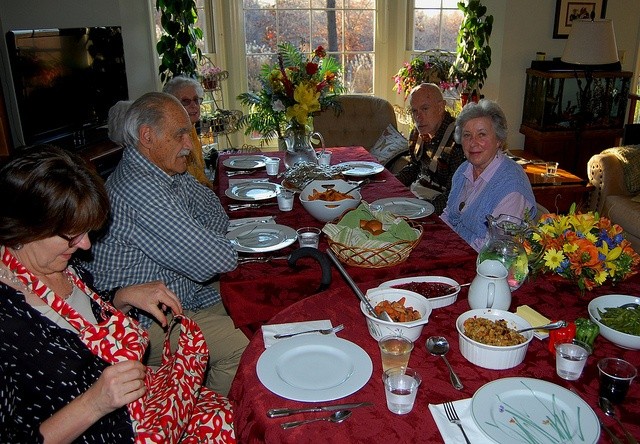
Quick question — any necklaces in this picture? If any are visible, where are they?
[0,269,74,298]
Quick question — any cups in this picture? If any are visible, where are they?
[265,157,281,176]
[316,151,333,165]
[277,189,295,212]
[295,226,321,249]
[553,338,592,381]
[378,335,415,376]
[546,161,559,177]
[381,366,423,416]
[597,358,638,416]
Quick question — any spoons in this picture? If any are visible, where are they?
[323,248,392,323]
[280,410,353,430]
[425,335,464,390]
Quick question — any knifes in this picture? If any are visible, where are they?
[239,257,289,261]
[267,402,364,419]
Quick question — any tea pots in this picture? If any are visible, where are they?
[475,214,548,293]
[467,258,512,310]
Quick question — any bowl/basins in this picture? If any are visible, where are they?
[455,308,534,370]
[587,294,640,351]
[359,288,433,342]
[300,179,362,224]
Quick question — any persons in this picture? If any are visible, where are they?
[394,82,464,214]
[0,146,184,444]
[162,77,213,189]
[70,93,251,398]
[439,100,537,252]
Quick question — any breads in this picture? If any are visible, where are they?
[359,219,385,235]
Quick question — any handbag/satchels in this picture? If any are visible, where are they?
[134,315,210,444]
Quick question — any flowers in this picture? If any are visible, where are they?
[395,48,469,100]
[202,67,221,77]
[519,203,640,291]
[235,39,341,146]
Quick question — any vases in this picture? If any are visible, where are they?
[202,76,218,89]
[282,120,323,169]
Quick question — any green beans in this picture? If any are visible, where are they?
[595,303,640,336]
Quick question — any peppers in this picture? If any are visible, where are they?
[547,319,577,357]
[574,317,600,352]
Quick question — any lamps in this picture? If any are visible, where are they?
[561,18,619,66]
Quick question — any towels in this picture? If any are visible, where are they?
[261,320,334,348]
[229,178,271,187]
[226,216,277,230]
[428,398,492,444]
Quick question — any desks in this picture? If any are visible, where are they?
[507,149,595,213]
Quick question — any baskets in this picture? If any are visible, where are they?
[325,208,423,268]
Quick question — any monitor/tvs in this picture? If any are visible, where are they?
[0,25,129,156]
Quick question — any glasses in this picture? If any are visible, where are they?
[57,223,97,249]
[181,98,203,107]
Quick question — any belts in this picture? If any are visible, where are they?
[418,177,446,193]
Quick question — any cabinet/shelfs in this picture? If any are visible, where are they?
[42,130,125,181]
[520,125,620,176]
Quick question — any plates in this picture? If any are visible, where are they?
[377,275,459,308]
[222,155,272,170]
[225,223,298,253]
[255,334,374,403]
[281,177,301,193]
[471,377,601,444]
[224,181,283,201]
[338,160,385,177]
[369,196,435,220]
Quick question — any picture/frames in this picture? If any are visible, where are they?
[552,0,606,39]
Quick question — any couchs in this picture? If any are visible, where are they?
[586,144,639,249]
[309,94,416,187]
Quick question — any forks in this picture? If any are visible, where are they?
[238,258,273,264]
[274,324,345,339]
[442,400,474,444]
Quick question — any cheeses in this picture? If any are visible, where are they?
[516,304,551,333]
